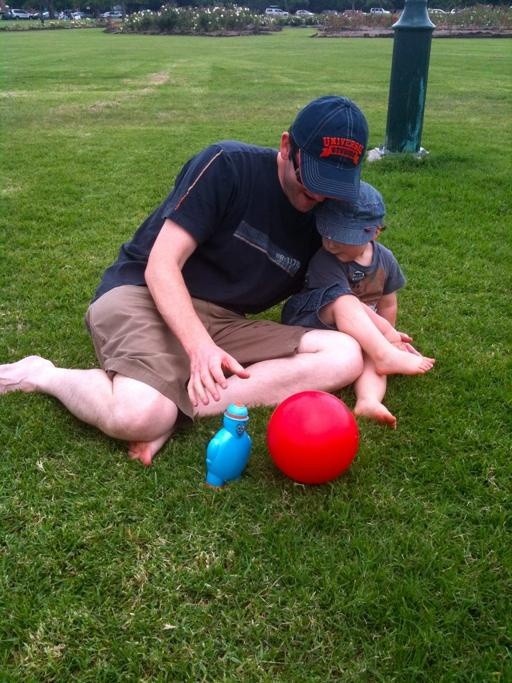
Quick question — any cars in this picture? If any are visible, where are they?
[31,9,123,21]
[427,8,461,15]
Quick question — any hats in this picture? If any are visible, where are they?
[317,180,385,246]
[290,95,370,202]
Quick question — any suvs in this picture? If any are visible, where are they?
[4,9,33,20]
[264,8,392,18]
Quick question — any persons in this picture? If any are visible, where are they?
[277,177,437,432]
[1,90,414,471]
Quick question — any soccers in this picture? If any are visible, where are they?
[267,390,360,484]
